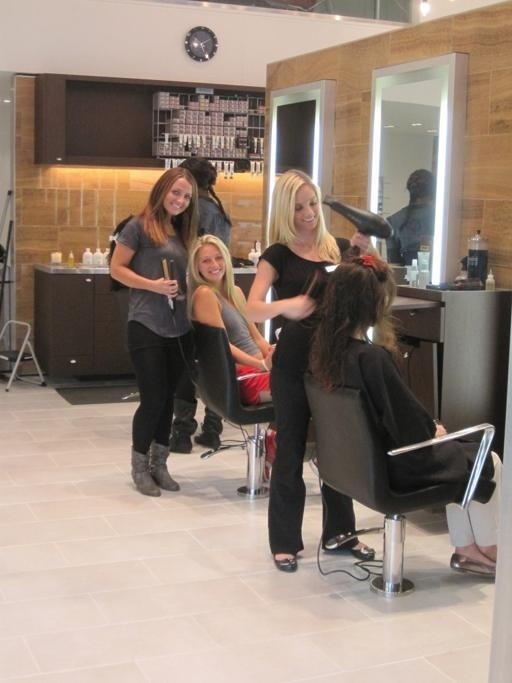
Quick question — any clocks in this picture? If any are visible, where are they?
[185,26,219,62]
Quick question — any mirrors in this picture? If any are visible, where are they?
[365,52,470,286]
[266,78,336,237]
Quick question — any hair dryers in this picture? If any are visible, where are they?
[323,193,395,240]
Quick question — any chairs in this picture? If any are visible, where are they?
[190,320,275,497]
[0,320,45,391]
[304,373,496,597]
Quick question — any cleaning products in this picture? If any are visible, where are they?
[417,251,431,282]
[82,247,110,266]
[67,250,75,268]
[467,230,487,289]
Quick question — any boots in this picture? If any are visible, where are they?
[169,396,197,453]
[149,440,181,490]
[194,407,223,451]
[131,447,164,496]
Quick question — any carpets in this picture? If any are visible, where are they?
[55,385,141,405]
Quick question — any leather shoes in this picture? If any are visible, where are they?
[449,553,496,575]
[349,542,376,561]
[273,554,296,572]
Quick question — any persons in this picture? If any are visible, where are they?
[385,169,434,264]
[168,157,231,453]
[110,168,199,496]
[187,233,277,480]
[246,169,382,572]
[308,255,503,580]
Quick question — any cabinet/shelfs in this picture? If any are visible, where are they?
[152,93,265,160]
[383,283,512,455]
[34,263,263,376]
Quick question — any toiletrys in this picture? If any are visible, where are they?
[50,251,63,264]
